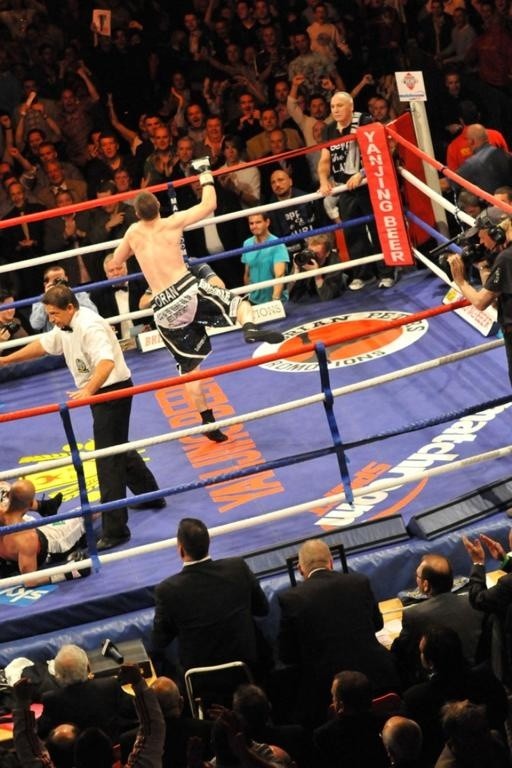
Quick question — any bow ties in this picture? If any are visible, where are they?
[112,284,129,292]
[60,325,74,333]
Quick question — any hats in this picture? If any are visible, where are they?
[465,206,510,239]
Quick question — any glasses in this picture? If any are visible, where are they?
[416,573,421,579]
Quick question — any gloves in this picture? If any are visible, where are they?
[49,550,93,585]
[188,155,215,187]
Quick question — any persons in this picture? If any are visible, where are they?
[286,231,343,302]
[0,289,29,342]
[447,208,512,392]
[233,686,312,768]
[461,528,512,753]
[0,91,84,220]
[185,704,295,767]
[119,676,215,768]
[0,481,102,588]
[0,284,167,550]
[457,189,488,218]
[30,266,99,332]
[45,724,80,768]
[138,257,225,310]
[367,95,383,114]
[269,169,315,237]
[12,663,167,768]
[306,120,326,183]
[371,98,392,126]
[115,155,284,443]
[38,643,140,742]
[398,626,510,748]
[241,213,289,306]
[317,92,402,291]
[277,539,384,668]
[0,182,48,293]
[0,0,407,91]
[381,716,423,768]
[312,672,390,768]
[149,518,269,669]
[286,73,374,147]
[390,554,483,662]
[91,254,156,340]
[41,190,96,287]
[84,91,294,200]
[493,186,512,214]
[87,180,138,312]
[433,700,512,768]
[407,0,512,193]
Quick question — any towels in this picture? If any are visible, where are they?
[343,111,363,175]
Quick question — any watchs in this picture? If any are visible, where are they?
[478,265,490,271]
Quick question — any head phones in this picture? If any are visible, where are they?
[480,208,507,245]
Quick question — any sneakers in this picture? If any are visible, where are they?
[39,491,64,518]
[129,496,168,511]
[347,274,379,291]
[95,527,132,551]
[198,421,228,443]
[377,266,401,290]
[242,329,286,344]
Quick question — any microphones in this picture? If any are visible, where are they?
[100,638,125,662]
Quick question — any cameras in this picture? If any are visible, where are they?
[2,321,23,337]
[53,277,69,290]
[428,226,488,275]
[293,249,319,268]
